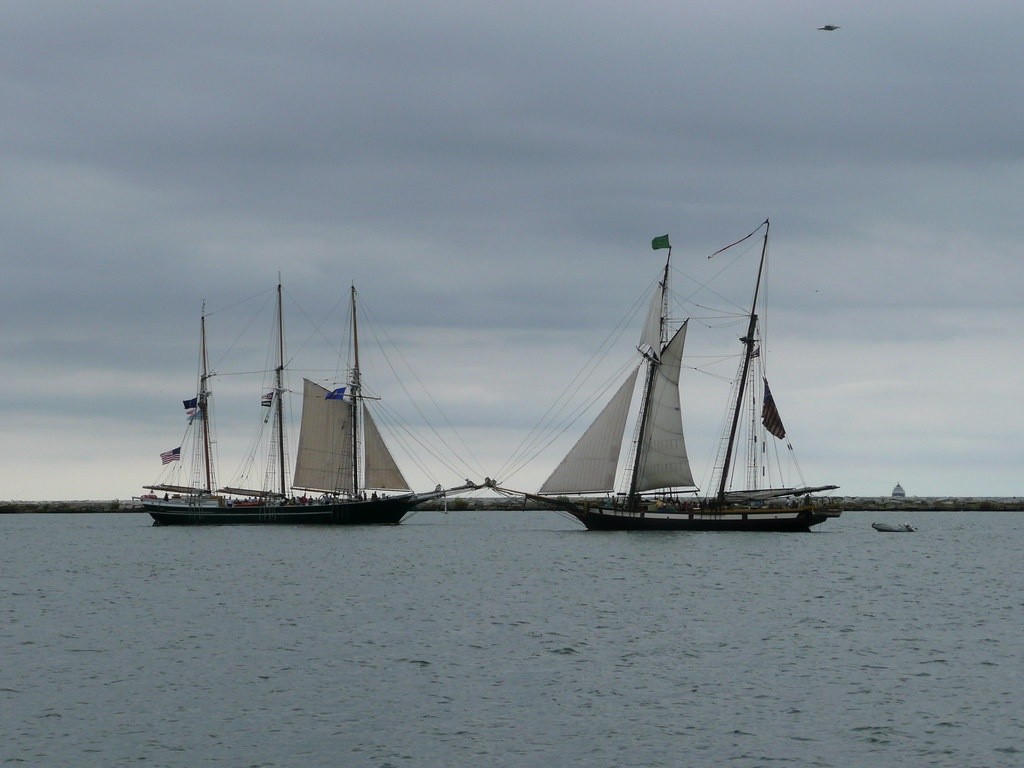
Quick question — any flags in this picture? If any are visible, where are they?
[760,377,786,440]
[261,391,273,407]
[160,447,181,464]
[325,387,347,401]
[651,234,671,250]
[183,398,205,421]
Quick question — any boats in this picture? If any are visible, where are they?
[871,519,919,533]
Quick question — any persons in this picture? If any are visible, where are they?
[583,495,639,514]
[653,494,718,511]
[226,491,378,507]
[786,493,816,508]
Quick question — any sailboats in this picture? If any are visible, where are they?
[130,269,490,527]
[491,219,845,534]
